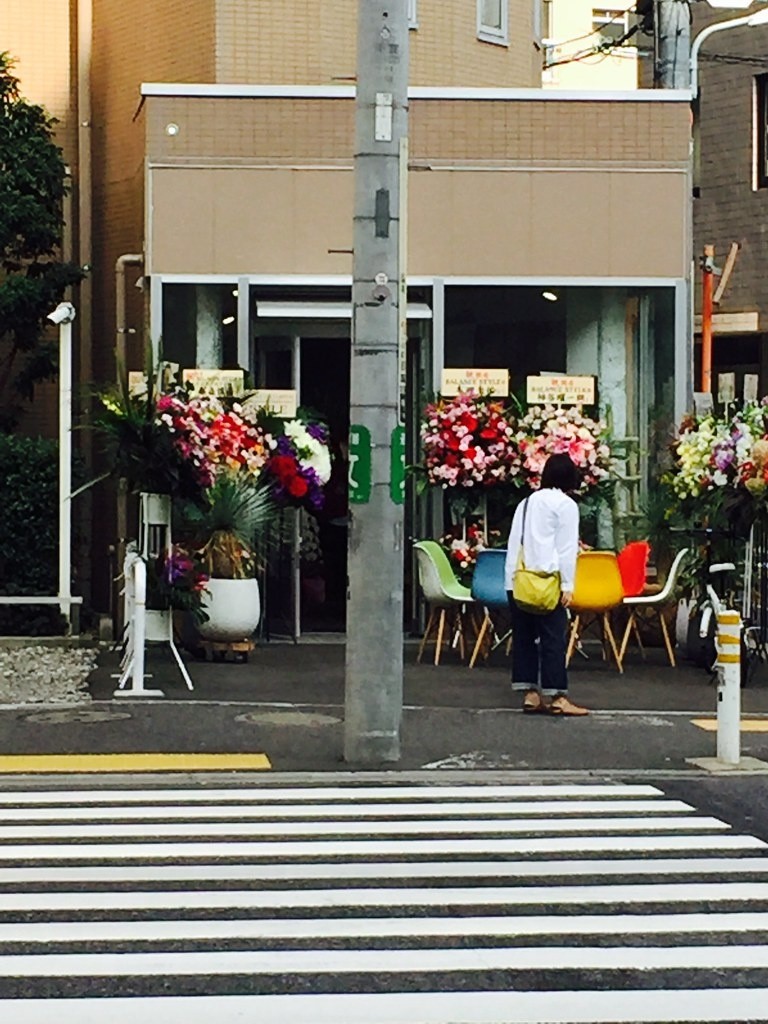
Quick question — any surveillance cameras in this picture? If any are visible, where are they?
[46,305,71,326]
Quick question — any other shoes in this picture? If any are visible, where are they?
[523,691,590,715]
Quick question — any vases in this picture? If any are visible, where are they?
[144,610,171,640]
[676,598,749,690]
[143,494,168,525]
[192,577,260,642]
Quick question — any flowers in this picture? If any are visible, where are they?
[66,336,332,628]
[660,396,768,596]
[419,386,611,570]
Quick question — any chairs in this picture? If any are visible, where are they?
[616,540,650,659]
[619,548,689,667]
[468,549,510,671]
[412,541,487,666]
[565,551,625,674]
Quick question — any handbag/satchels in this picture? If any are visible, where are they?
[512,545,562,615]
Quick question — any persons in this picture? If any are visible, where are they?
[504,453,593,716]
